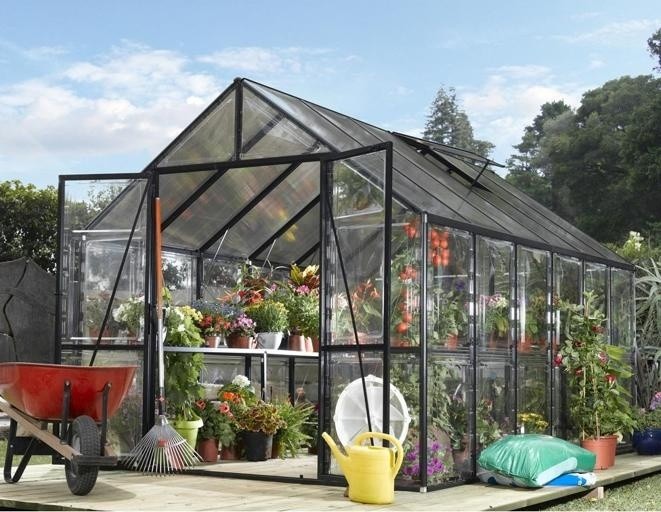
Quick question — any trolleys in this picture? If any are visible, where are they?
[0,362,141,495]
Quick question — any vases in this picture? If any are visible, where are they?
[193,430,289,460]
[631,427,661,457]
[580,437,619,467]
[203,332,368,358]
[89,329,141,347]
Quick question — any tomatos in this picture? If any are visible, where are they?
[394,217,449,333]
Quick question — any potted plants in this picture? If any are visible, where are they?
[154,305,204,465]
[428,289,571,353]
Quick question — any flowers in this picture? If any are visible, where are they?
[630,392,661,426]
[195,374,287,429]
[399,434,449,487]
[86,261,382,333]
[550,288,623,435]
